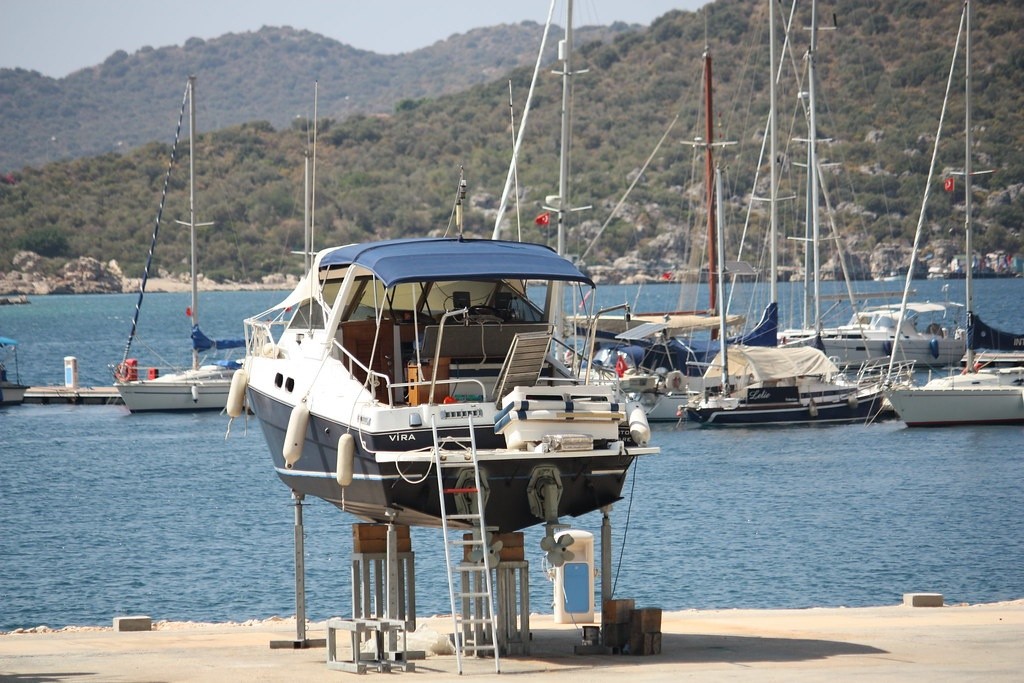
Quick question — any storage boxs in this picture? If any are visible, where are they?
[602,597,662,656]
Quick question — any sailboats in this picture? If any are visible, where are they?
[463,0,897,426]
[886,0,1024,424]
[108,75,293,412]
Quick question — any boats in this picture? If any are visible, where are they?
[244,166,661,535]
[779,296,965,366]
[0,337,30,404]
